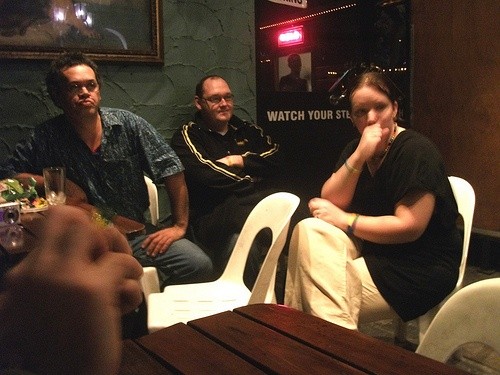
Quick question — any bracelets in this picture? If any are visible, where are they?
[226,155,231,167]
[347,212,359,235]
[344,160,362,174]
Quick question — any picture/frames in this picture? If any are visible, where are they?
[0,0,164,63]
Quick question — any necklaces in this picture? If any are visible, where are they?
[378,121,397,158]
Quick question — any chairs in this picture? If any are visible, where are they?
[415,278,500,363]
[394,175,476,347]
[147,192,300,334]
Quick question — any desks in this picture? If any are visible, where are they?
[118,303,474,375]
[0,194,146,268]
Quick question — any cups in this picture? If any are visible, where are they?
[42,167,67,206]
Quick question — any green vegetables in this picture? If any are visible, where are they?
[2,177,37,202]
[100,207,117,223]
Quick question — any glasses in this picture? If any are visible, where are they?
[200,93,235,105]
[59,81,99,95]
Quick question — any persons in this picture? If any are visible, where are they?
[171,75,303,304]
[0,204,144,375]
[284,72,464,332]
[0,53,213,293]
